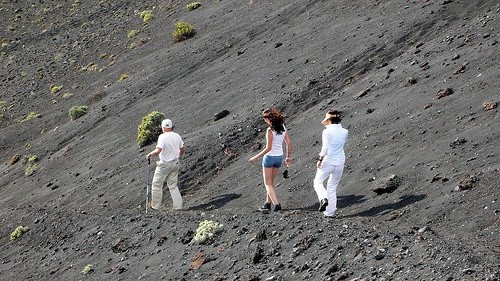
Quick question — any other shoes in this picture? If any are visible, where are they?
[324,214,335,218]
[148,202,152,208]
[318,198,328,212]
[172,208,182,211]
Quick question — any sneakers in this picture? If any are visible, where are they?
[273,204,283,213]
[259,202,271,211]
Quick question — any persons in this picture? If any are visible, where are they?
[146,119,184,211]
[313,110,349,217]
[248,107,291,212]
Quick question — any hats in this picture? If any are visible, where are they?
[161,119,172,128]
[321,109,340,125]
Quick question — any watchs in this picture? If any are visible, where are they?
[316,158,322,161]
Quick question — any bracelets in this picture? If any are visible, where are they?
[256,154,259,159]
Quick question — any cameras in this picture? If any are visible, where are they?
[283,170,288,178]
